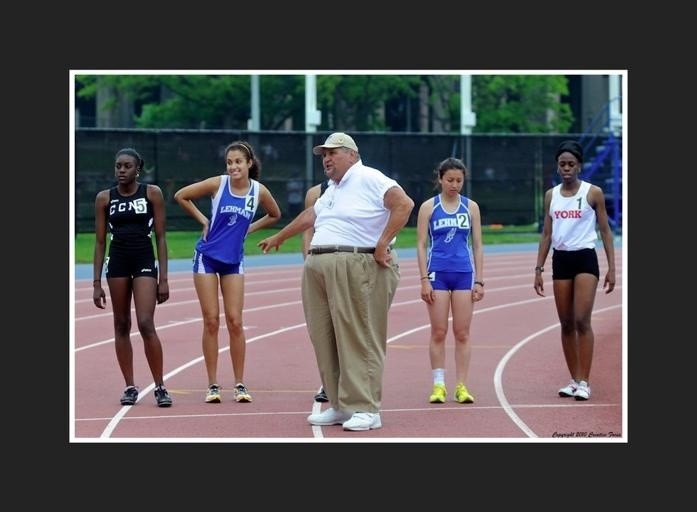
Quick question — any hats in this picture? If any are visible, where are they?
[312,133,358,153]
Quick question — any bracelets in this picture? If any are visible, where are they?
[419,276,431,281]
[93,279,101,283]
[473,280,483,287]
[535,265,544,274]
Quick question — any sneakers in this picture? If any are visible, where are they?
[120,386,139,405]
[429,383,447,403]
[233,384,252,402]
[307,406,354,430]
[314,386,329,402]
[453,383,474,403]
[343,411,383,430]
[204,383,222,403]
[558,379,593,400]
[154,385,172,407]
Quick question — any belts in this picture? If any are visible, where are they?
[307,245,394,254]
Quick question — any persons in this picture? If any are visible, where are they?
[174,139,280,405]
[532,146,615,400]
[91,144,172,408]
[256,132,413,431]
[416,156,483,403]
[302,179,331,407]
